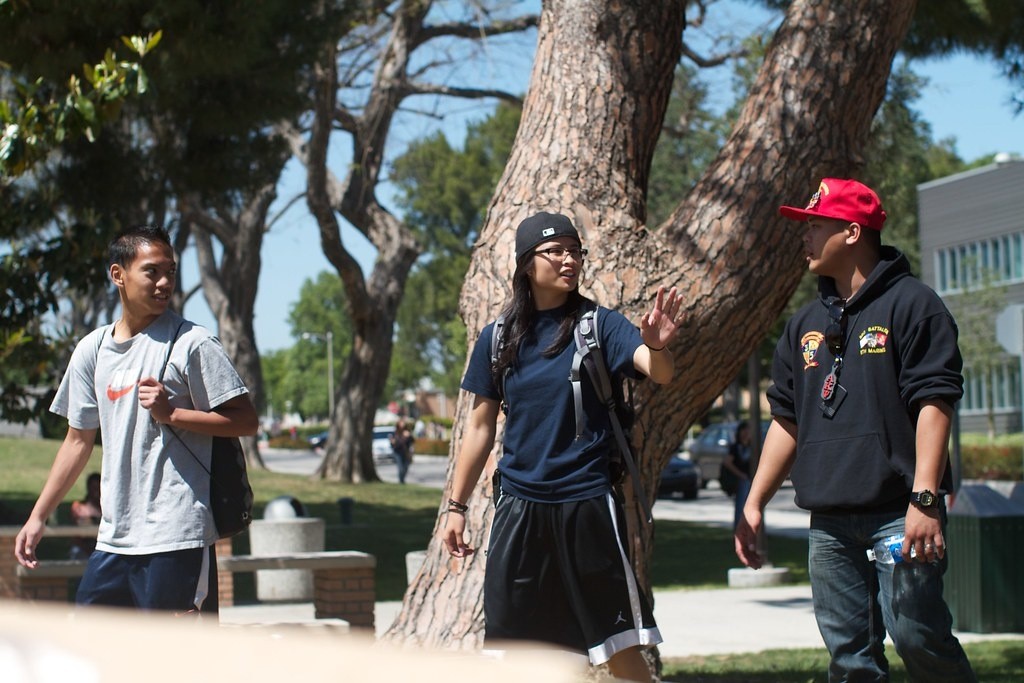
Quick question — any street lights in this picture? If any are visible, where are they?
[301,330,336,429]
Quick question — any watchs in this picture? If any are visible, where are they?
[911,490,940,509]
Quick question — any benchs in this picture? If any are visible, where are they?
[0,527,234,607]
[16,550,376,638]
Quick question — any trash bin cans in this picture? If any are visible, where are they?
[939,483,1024,632]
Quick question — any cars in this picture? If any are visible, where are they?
[655,455,701,500]
[371,425,415,464]
[687,420,771,490]
[306,429,329,452]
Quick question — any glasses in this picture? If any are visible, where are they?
[824,299,847,353]
[533,245,587,263]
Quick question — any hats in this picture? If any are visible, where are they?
[515,211,582,261]
[779,179,888,232]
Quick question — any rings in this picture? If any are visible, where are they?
[924,544,933,549]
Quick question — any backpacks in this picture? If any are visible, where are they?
[158,321,254,539]
[718,444,743,495]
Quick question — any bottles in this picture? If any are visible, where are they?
[866,534,944,565]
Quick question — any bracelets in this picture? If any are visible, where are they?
[448,498,468,513]
[645,342,665,351]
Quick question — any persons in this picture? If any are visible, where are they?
[724,419,751,525]
[388,419,415,483]
[735,178,973,683]
[15,227,259,616]
[443,211,684,683]
